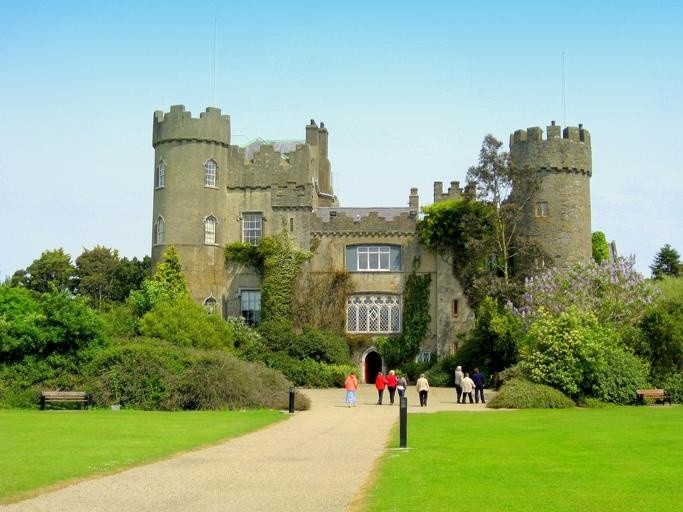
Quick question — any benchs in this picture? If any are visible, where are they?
[635,388,673,406]
[39,390,93,411]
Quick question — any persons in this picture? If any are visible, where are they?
[472,368,486,404]
[462,373,476,404]
[415,373,430,407]
[344,371,358,408]
[387,370,407,405]
[454,365,464,404]
[375,372,388,405]
